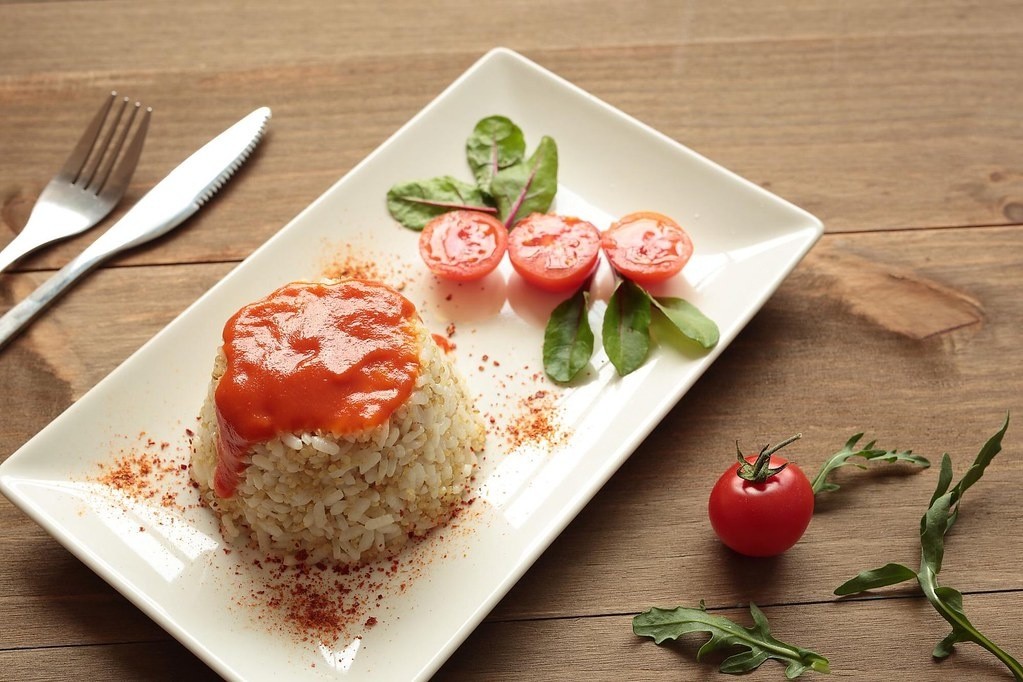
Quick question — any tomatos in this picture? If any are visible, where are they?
[599,209,694,286]
[707,435,816,558]
[507,214,600,291]
[420,209,508,284]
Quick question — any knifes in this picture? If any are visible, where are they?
[0,103,274,357]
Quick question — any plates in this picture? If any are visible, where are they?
[1,44,823,682]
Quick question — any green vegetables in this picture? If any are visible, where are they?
[631,599,829,679]
[542,280,719,382]
[832,413,1023,682]
[386,115,557,233]
[813,431,928,505]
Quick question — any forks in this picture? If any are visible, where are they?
[0,89,154,281]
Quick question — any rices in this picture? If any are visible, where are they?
[189,280,487,568]
